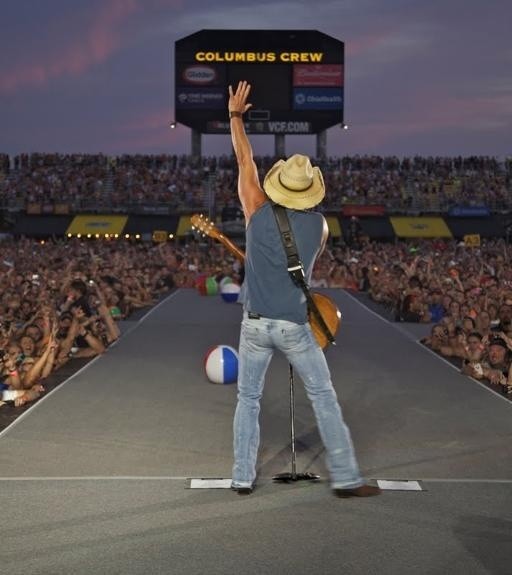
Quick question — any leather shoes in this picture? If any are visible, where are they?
[336,485,381,498]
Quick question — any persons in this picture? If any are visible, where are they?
[226,80,384,499]
[1,152,246,409]
[253,154,510,390]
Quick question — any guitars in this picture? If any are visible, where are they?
[190,214,341,353]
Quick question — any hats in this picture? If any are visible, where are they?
[262,153,326,211]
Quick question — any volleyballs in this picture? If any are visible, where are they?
[204,345,239,384]
[199,278,217,296]
[222,283,241,302]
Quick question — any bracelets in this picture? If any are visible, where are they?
[11,370,17,376]
[83,330,89,338]
[227,109,245,121]
[464,346,469,351]
[10,368,16,372]
[479,344,484,350]
